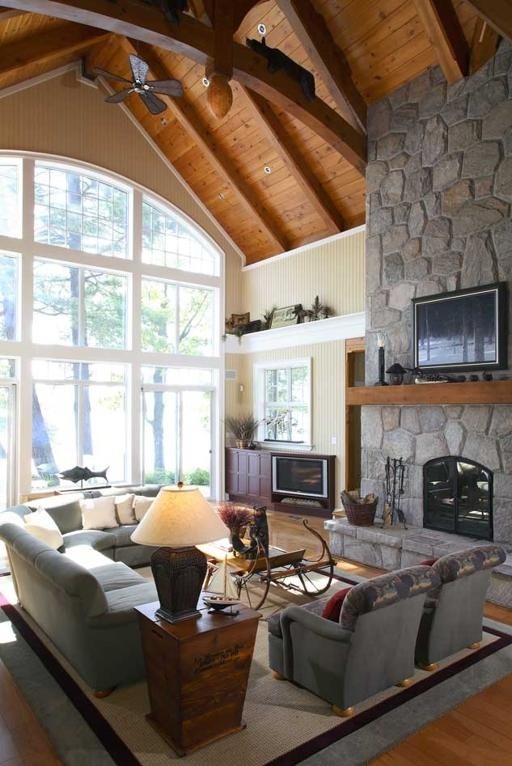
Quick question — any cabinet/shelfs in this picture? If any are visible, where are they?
[248,449,273,506]
[226,448,247,510]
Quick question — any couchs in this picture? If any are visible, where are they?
[1,484,176,697]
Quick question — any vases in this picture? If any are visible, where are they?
[229,526,241,544]
[234,439,248,449]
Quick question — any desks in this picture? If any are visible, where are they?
[135,590,263,755]
[190,517,338,610]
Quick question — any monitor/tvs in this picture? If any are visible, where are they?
[410,280,509,375]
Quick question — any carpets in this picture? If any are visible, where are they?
[1,557,512,766]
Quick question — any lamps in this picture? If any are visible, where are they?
[132,480,233,623]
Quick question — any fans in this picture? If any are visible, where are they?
[85,41,184,114]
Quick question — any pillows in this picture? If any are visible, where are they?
[80,496,120,530]
[25,504,43,522]
[113,493,139,524]
[131,495,155,524]
[320,587,353,622]
[27,509,64,550]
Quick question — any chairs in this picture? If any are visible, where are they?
[268,567,440,717]
[411,545,507,671]
